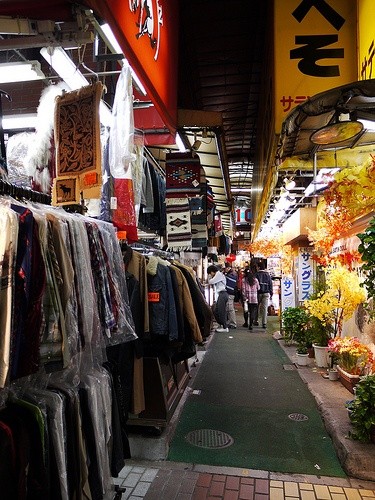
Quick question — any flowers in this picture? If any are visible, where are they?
[326,335,375,377]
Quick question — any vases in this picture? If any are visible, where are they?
[326,369,338,382]
[335,364,364,395]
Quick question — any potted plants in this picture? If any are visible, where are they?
[343,373,375,444]
[281,271,337,368]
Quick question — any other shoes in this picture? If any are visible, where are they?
[230,324,235,328]
[249,326,253,330]
[215,325,229,333]
[242,323,248,327]
[262,324,266,328]
[253,322,258,325]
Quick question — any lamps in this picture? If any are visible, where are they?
[283,177,296,190]
[193,131,202,150]
[280,186,289,198]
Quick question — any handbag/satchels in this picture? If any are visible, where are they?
[234,286,241,302]
[243,299,250,313]
[267,299,275,315]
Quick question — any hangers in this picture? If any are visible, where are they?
[0,57,177,401]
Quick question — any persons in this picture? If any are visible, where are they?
[234,268,242,304]
[242,262,274,330]
[207,265,229,332]
[224,264,237,328]
